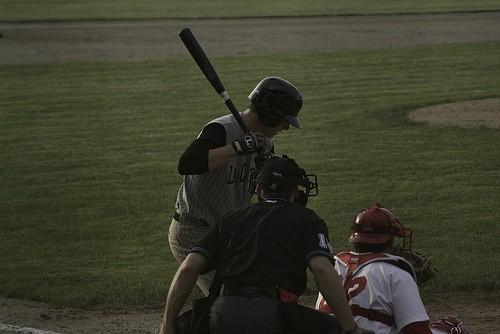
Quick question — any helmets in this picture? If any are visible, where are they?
[248,76,304,129]
[347,206,406,244]
[253,155,318,206]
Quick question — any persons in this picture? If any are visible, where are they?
[169,77,303,296]
[159,157,378,334]
[314,207,464,334]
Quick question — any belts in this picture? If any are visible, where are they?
[172,211,206,225]
[222,285,274,297]
[232,132,276,158]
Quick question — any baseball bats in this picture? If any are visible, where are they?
[177,27,264,162]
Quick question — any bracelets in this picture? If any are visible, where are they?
[344,324,358,334]
